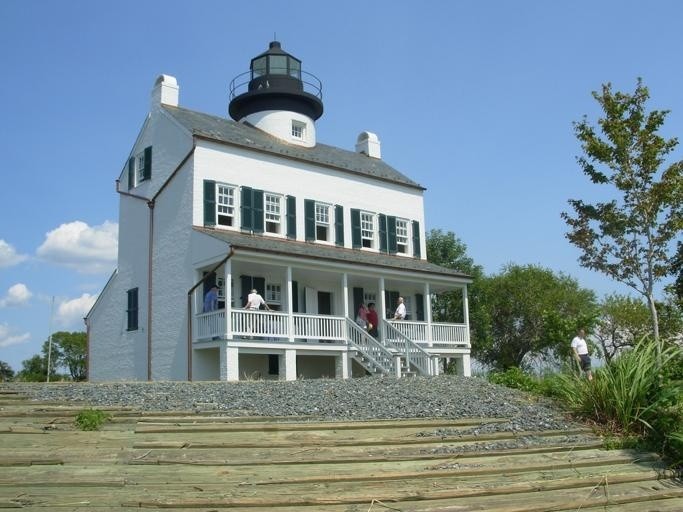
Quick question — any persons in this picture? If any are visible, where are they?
[388,296,406,321]
[568,328,593,381]
[202,284,226,342]
[241,288,270,340]
[355,302,369,331]
[366,302,379,341]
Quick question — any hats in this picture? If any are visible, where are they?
[210,285,220,289]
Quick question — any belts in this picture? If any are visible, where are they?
[249,307,259,310]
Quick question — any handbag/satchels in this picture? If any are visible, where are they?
[356,313,367,326]
